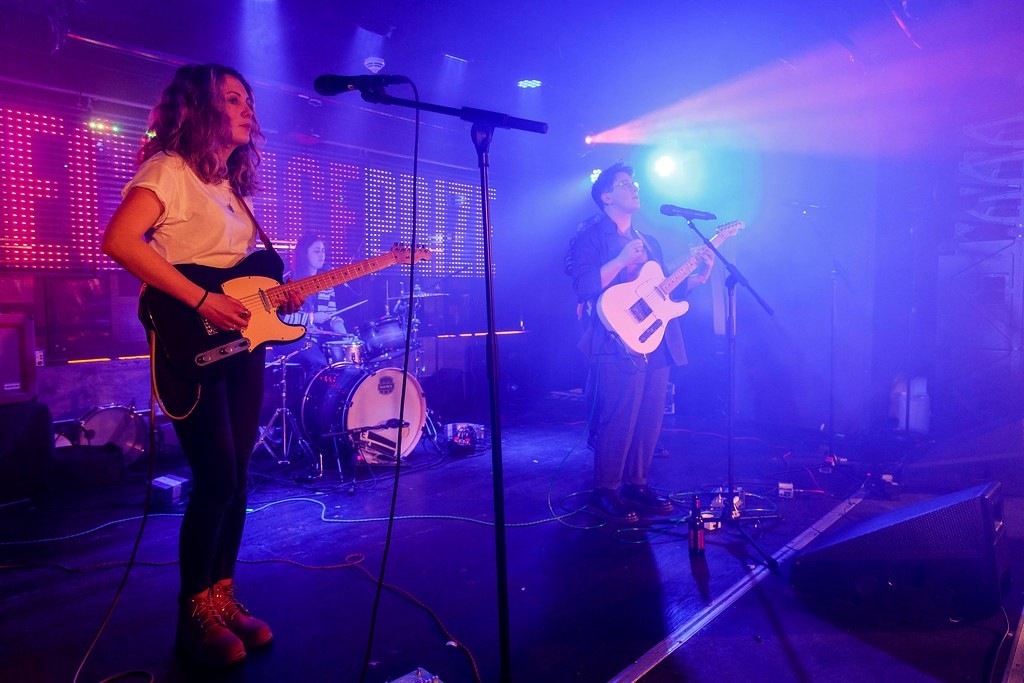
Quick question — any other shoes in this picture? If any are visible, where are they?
[622,486,673,513]
[654,448,670,458]
[585,436,597,451]
[584,487,639,523]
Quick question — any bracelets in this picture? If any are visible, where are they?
[194,290,208,310]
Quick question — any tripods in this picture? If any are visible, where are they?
[251,332,321,475]
[782,211,879,496]
[639,218,782,569]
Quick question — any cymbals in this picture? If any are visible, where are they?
[305,328,344,336]
[386,291,451,300]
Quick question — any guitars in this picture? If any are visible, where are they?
[595,219,745,358]
[136,241,433,379]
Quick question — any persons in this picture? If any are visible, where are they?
[281,234,348,379]
[563,159,714,524]
[99,62,307,660]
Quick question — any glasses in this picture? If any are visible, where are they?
[607,180,640,193]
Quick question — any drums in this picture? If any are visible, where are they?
[322,339,367,364]
[352,315,421,363]
[68,401,149,470]
[301,361,427,465]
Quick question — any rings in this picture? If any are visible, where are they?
[228,323,237,330]
[241,309,248,317]
[635,248,640,253]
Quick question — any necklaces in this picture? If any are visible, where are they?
[215,181,235,213]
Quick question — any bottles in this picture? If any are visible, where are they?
[688,494,705,556]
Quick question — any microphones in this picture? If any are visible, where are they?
[314,73,409,96]
[660,204,717,220]
[793,201,825,209]
[386,418,410,428]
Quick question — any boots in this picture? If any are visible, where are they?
[181,586,245,664]
[213,578,273,645]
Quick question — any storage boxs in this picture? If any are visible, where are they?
[663,383,677,415]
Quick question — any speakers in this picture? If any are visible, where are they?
[795,479,1010,633]
[889,411,1024,499]
[0,313,39,405]
